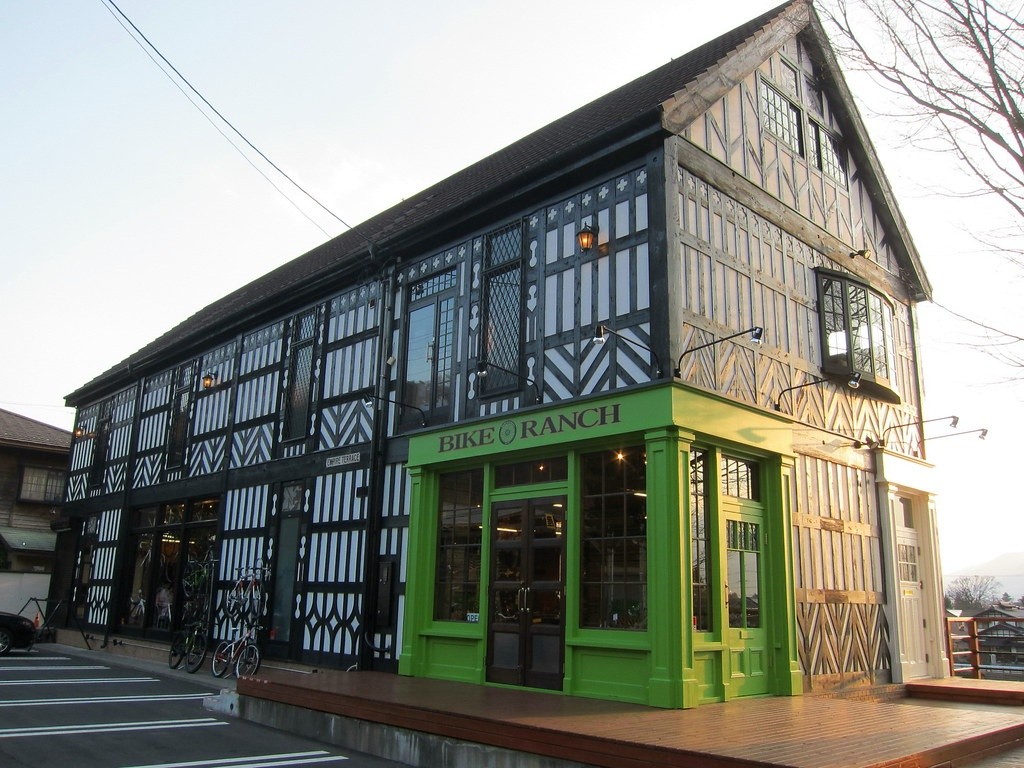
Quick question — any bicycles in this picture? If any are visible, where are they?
[212,623,271,679]
[128,538,205,626]
[168,618,213,673]
[225,566,262,620]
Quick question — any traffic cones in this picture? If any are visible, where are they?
[34,613,40,627]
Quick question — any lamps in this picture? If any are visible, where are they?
[914,428,987,449]
[850,249,872,259]
[592,323,660,381]
[854,436,880,450]
[202,372,215,389]
[576,220,596,253]
[776,372,862,411]
[883,416,962,447]
[361,391,428,427]
[677,326,764,378]
[477,359,544,407]
[74,428,83,439]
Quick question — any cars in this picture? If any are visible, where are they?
[0,612,36,656]
